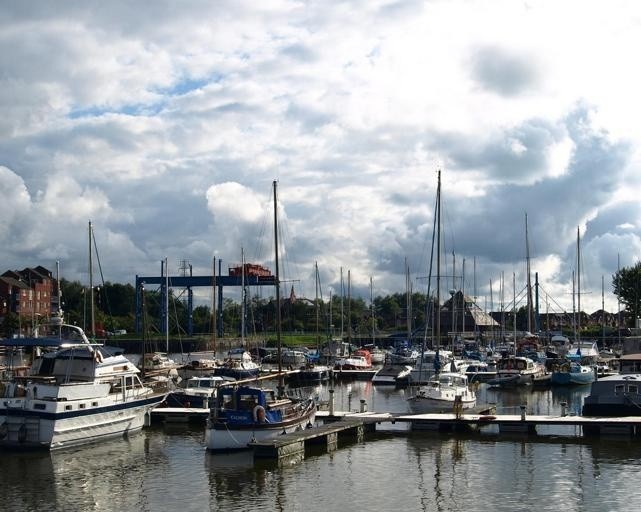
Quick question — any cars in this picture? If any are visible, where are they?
[611,328,632,337]
